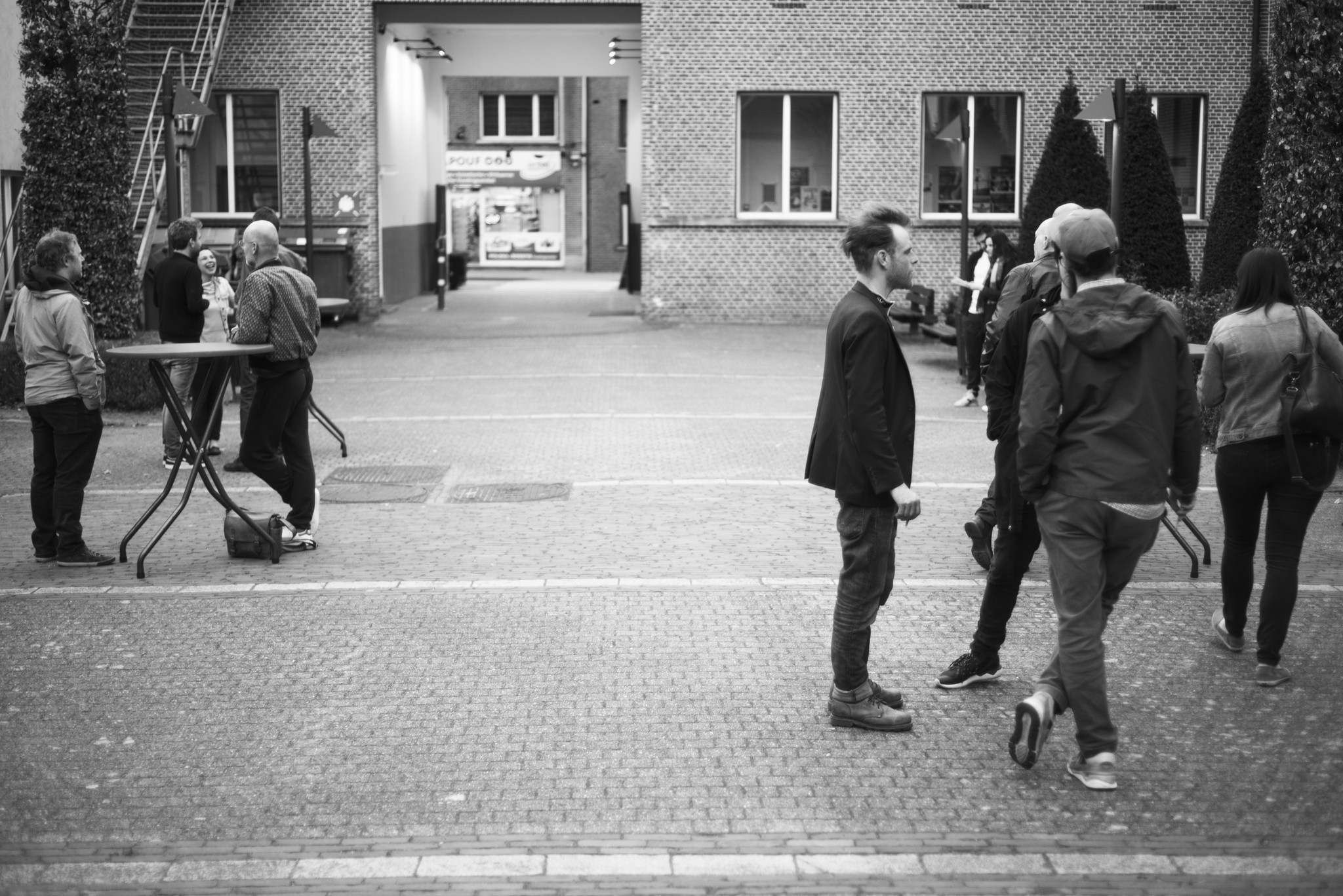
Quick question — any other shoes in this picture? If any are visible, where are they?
[981,405,988,413]
[964,517,993,570]
[208,441,221,454]
[224,456,252,473]
[281,529,312,545]
[1254,665,1291,685]
[310,488,320,536]
[954,392,975,407]
[1211,606,1245,651]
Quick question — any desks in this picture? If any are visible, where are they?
[1163,344,1211,577]
[306,297,351,458]
[106,342,280,579]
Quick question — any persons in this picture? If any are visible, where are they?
[1007,203,1204,788]
[223,205,308,472]
[1196,248,1343,685]
[153,216,206,469]
[969,230,1017,413]
[191,244,237,456]
[954,223,995,406]
[935,243,1067,689]
[15,230,116,567]
[804,202,920,731]
[231,220,321,545]
[964,217,1059,571]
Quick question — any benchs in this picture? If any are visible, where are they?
[889,284,937,333]
[917,293,958,345]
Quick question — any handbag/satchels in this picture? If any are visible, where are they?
[224,507,297,558]
[1278,306,1343,444]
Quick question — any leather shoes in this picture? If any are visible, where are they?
[830,679,904,710]
[830,697,913,731]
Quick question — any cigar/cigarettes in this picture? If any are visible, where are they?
[905,520,909,526]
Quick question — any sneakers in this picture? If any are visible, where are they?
[1067,750,1118,789]
[1009,690,1055,768]
[36,544,59,562]
[57,548,116,568]
[163,451,206,469]
[935,653,1004,688]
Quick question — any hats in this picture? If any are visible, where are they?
[1050,203,1117,268]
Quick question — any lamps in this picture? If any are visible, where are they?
[406,46,444,56]
[609,55,641,65]
[608,37,642,48]
[416,54,452,61]
[394,37,435,46]
[609,47,641,57]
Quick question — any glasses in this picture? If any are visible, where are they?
[238,240,259,250]
[1057,256,1065,267]
